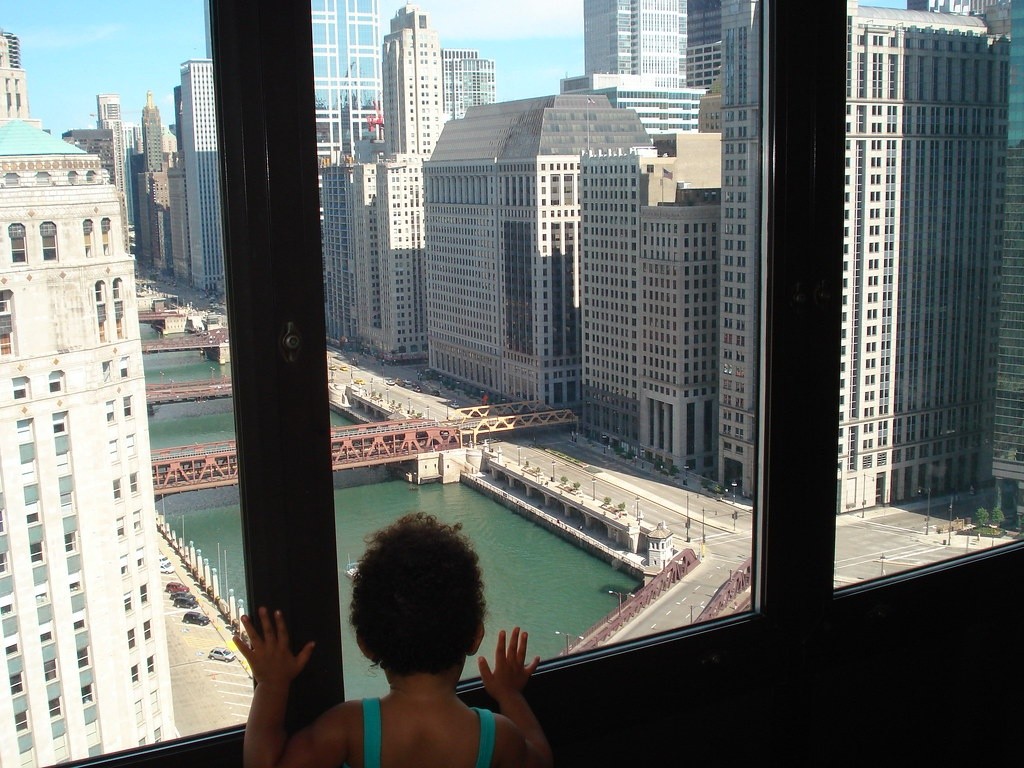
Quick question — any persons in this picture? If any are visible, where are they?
[232,512,555,768]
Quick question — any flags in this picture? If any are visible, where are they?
[663,169,672,180]
[588,98,595,104]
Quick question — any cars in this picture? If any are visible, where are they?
[166,582,190,594]
[172,597,198,610]
[208,647,236,663]
[159,555,172,562]
[159,561,175,574]
[182,611,211,626]
[169,591,196,601]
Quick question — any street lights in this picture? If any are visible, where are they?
[918,486,931,535]
[555,631,584,655]
[609,590,635,617]
[861,474,874,518]
[880,553,885,576]
[676,602,705,623]
[327,346,738,557]
